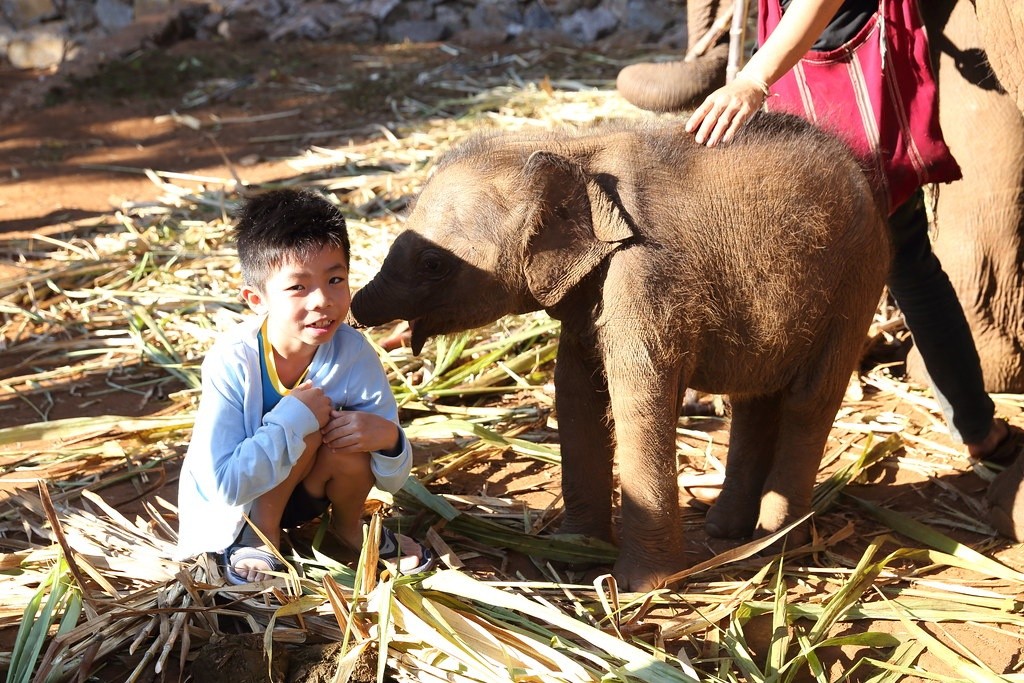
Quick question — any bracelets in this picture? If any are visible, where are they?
[738,72,770,96]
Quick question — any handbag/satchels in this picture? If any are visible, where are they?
[758,0,963,219]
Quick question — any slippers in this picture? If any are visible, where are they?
[992,416,1024,464]
[363,520,434,576]
[225,543,277,586]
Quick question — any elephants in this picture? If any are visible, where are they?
[342,1,1024,594]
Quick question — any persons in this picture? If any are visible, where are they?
[177,189,423,590]
[686,0,1024,478]
